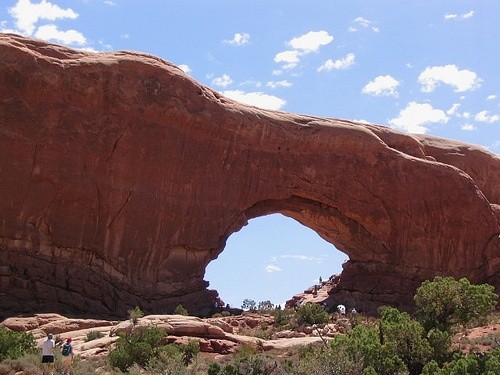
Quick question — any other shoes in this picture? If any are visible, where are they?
[61,372,71,375]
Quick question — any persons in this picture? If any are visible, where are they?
[40,333,61,375]
[60,337,75,375]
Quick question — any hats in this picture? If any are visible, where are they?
[66,338,72,342]
[48,333,53,339]
